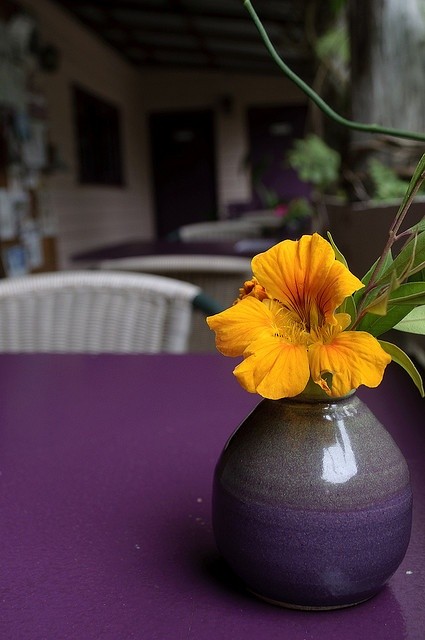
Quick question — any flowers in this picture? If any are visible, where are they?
[203,223,424,403]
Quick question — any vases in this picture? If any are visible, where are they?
[211,377,414,610]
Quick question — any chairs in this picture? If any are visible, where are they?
[1,270,198,355]
[97,256,255,353]
[179,219,262,240]
